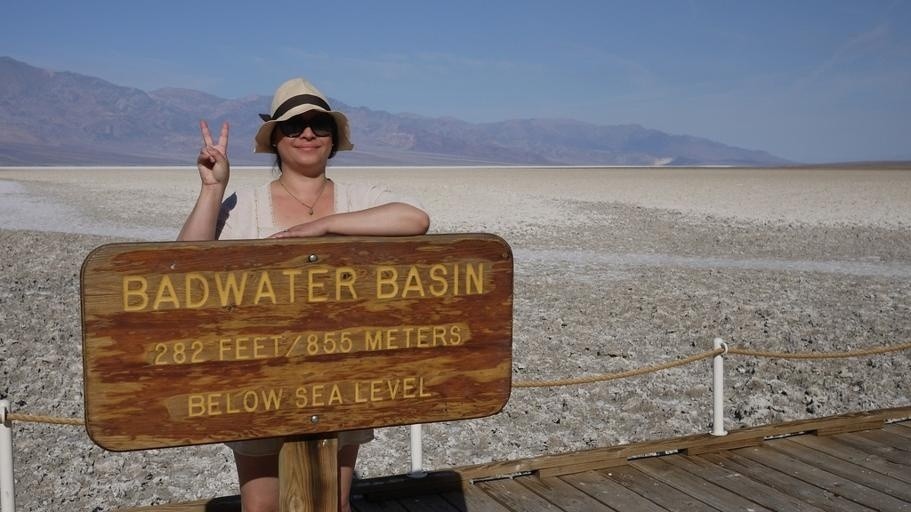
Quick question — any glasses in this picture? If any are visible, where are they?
[275,114,334,138]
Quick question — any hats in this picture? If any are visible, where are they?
[254,77,355,151]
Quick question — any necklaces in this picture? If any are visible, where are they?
[275,177,329,216]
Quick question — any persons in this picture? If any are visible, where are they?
[176,75,432,511]
